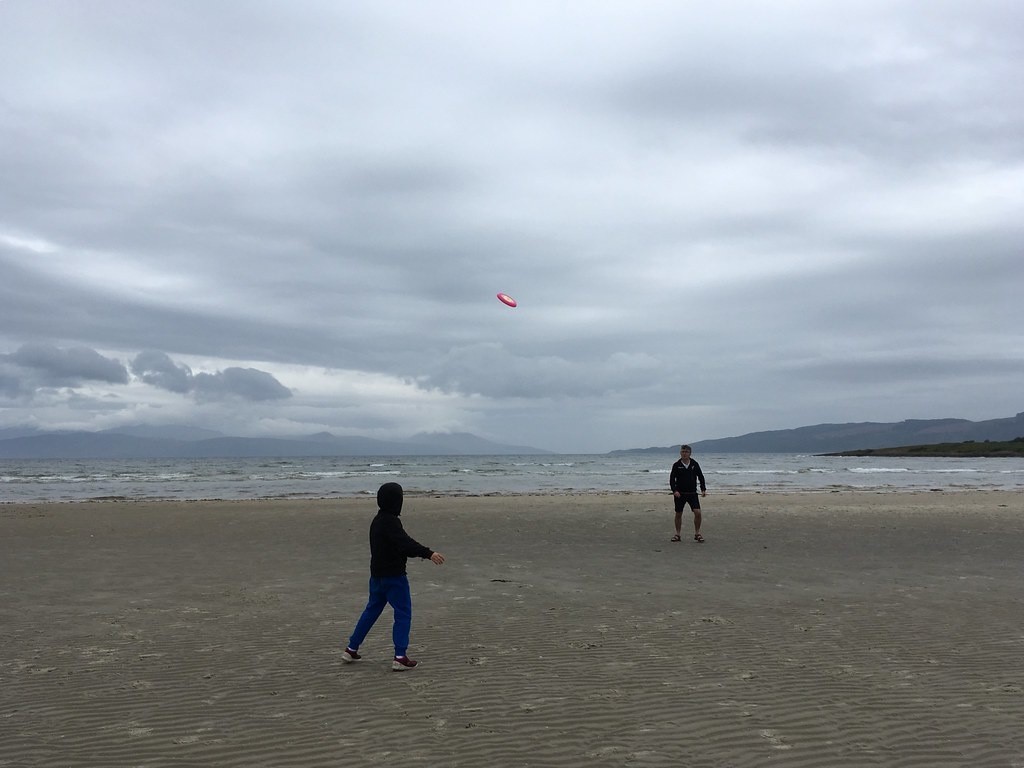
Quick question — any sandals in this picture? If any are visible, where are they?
[671,535,681,541]
[694,533,704,541]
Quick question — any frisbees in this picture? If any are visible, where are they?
[497,293,516,307]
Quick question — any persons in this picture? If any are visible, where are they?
[669,445,707,544]
[342,483,444,671]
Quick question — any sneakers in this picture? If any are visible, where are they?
[342,647,362,661]
[391,655,418,670]
